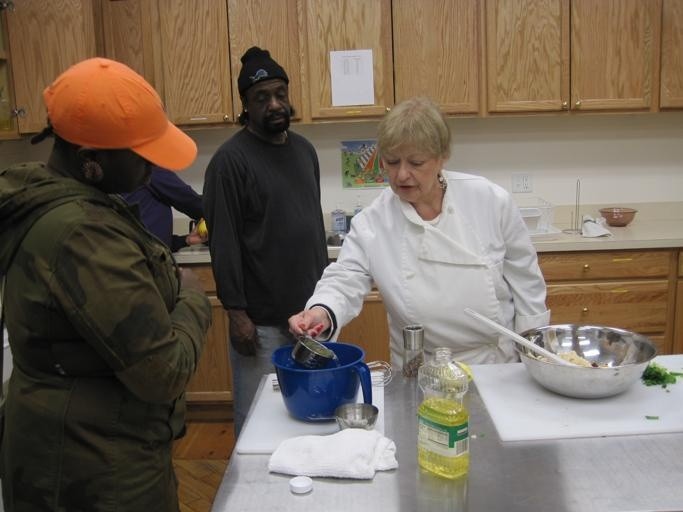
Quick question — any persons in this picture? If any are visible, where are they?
[287,94,551,370]
[119,164,203,249]
[203,46,329,441]
[0,57,213,512]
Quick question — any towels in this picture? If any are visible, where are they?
[262,421,400,483]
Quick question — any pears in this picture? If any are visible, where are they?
[198,220,208,239]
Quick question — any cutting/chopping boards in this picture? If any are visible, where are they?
[465,354,682,443]
[234,368,385,457]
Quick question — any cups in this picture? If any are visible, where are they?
[270,342,372,424]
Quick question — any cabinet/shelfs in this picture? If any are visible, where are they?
[0,1,157,144]
[298,1,485,126]
[149,1,302,131]
[479,1,682,121]
[164,246,682,426]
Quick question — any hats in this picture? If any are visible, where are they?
[41,54,201,173]
[235,44,290,96]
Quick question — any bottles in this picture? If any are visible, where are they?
[414,346,471,481]
[401,323,426,379]
[329,205,362,233]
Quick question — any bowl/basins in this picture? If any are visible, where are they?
[515,208,542,233]
[510,324,658,401]
[598,207,637,228]
